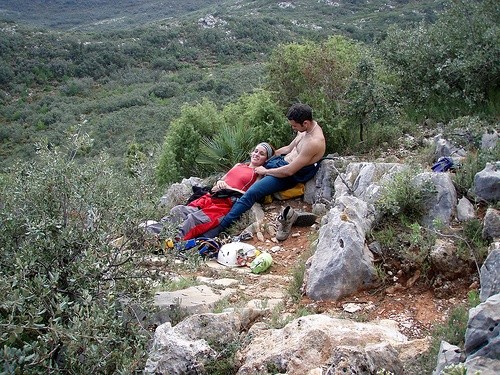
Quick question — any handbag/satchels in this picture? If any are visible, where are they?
[431,157,466,172]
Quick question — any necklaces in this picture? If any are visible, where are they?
[306,121,317,134]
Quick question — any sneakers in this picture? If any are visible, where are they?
[282,208,316,226]
[276,205,298,241]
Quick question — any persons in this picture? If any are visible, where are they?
[192,103,325,238]
[126,142,275,251]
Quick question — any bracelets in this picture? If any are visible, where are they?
[216,179,220,183]
